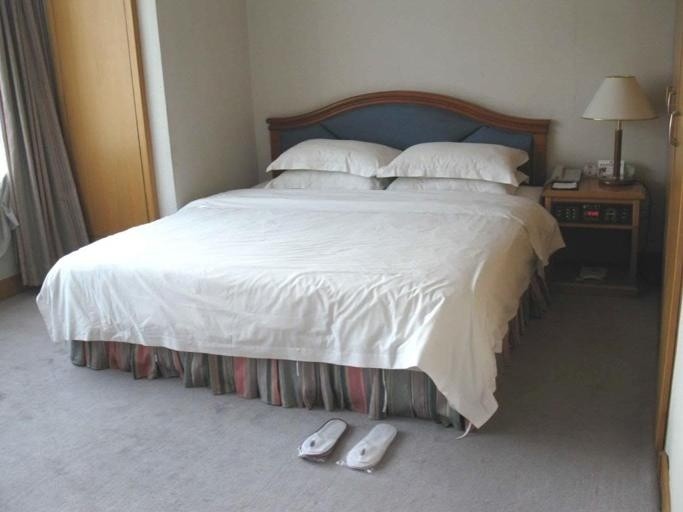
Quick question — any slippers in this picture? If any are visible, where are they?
[345,421,398,471]
[299,417,349,461]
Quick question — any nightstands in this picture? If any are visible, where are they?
[540,174,647,300]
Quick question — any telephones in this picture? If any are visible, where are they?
[552,165,582,182]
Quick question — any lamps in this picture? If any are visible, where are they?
[579,74,663,191]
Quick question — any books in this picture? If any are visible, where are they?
[544,165,583,190]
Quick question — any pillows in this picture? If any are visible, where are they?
[377,139,530,190]
[263,137,402,179]
[260,169,391,191]
[383,170,530,196]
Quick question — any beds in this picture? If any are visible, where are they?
[32,88,572,444]
